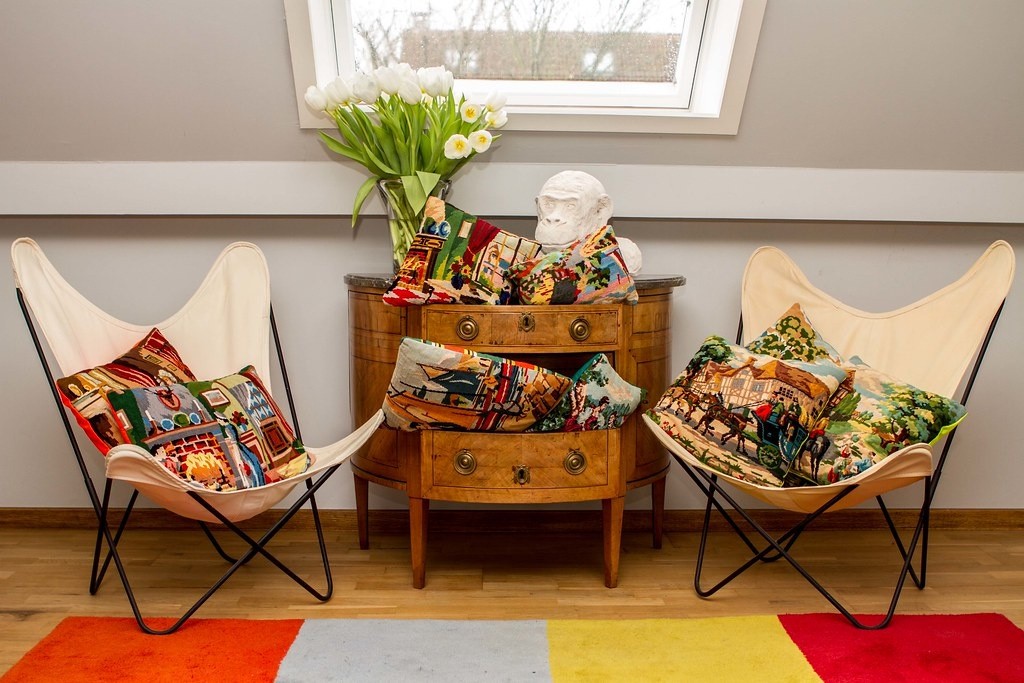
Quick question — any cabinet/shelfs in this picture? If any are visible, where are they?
[375,178,451,278]
[344,272,689,590]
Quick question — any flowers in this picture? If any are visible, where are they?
[303,62,508,272]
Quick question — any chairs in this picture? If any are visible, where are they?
[642,240,1015,631]
[10,236,387,635]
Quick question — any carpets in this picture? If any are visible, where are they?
[0,611,1024,683]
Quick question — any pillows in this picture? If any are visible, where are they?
[379,194,542,308]
[525,352,649,433]
[104,364,317,490]
[380,336,573,432]
[743,303,848,489]
[505,224,639,307]
[790,355,969,487]
[645,334,847,488]
[55,326,199,457]
